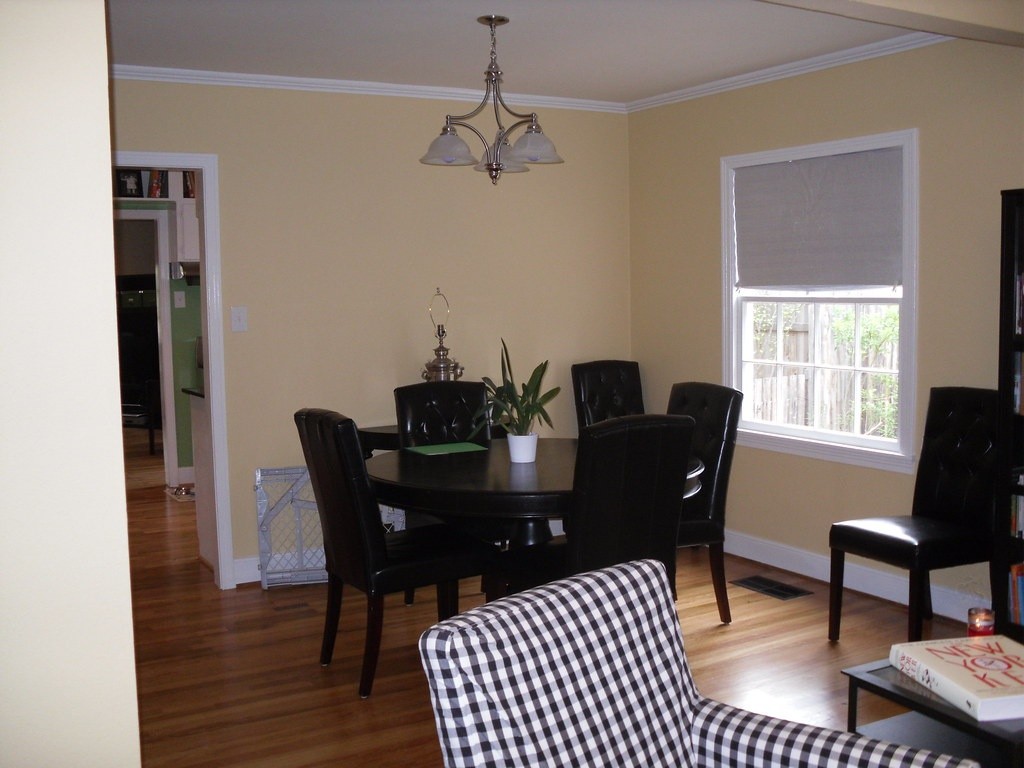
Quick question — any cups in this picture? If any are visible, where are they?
[968,607,995,638]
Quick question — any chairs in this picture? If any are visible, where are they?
[293,407,459,699]
[486,414,695,602]
[393,381,490,607]
[418,558,982,768]
[666,381,744,625]
[828,388,1015,642]
[571,360,645,434]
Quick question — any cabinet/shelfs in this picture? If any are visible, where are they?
[990,188,1024,642]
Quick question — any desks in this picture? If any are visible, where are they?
[365,438,706,596]
[840,658,1024,768]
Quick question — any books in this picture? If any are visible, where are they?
[887,635,1024,722]
[1009,273,1024,627]
[116,168,143,197]
[147,170,196,198]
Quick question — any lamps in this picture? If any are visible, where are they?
[419,15,565,186]
[465,337,562,463]
